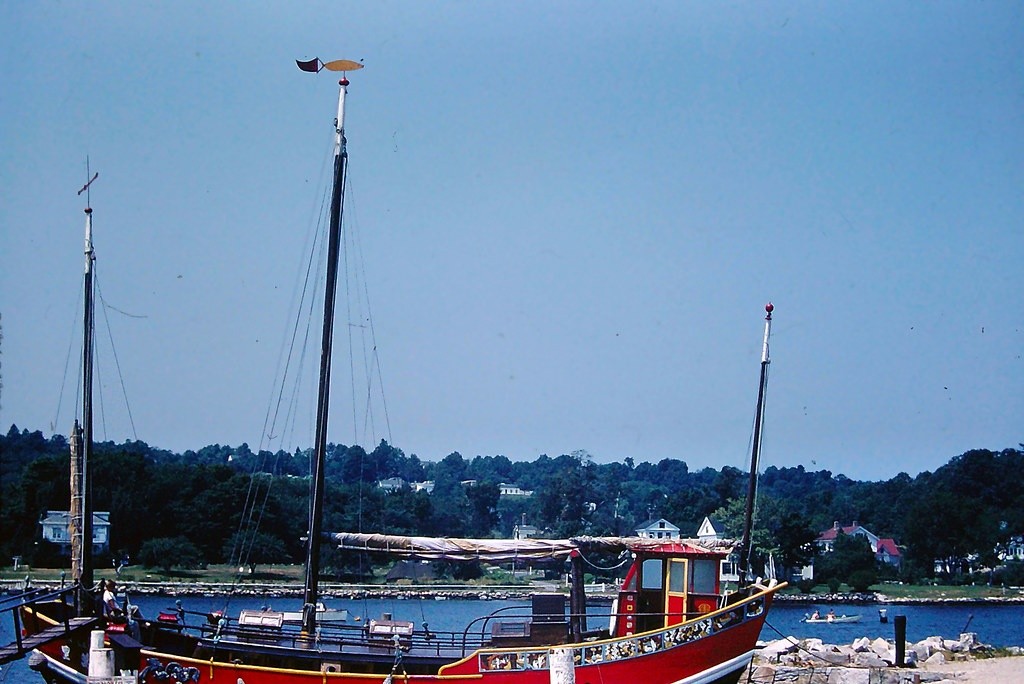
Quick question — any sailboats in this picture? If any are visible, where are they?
[17,56,789,684]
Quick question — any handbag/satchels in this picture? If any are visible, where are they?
[107,609,128,624]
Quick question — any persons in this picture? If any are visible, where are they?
[825,609,836,619]
[103,581,140,643]
[811,610,820,620]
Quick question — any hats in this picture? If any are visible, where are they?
[106,579,118,587]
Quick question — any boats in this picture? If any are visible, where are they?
[806,613,864,624]
